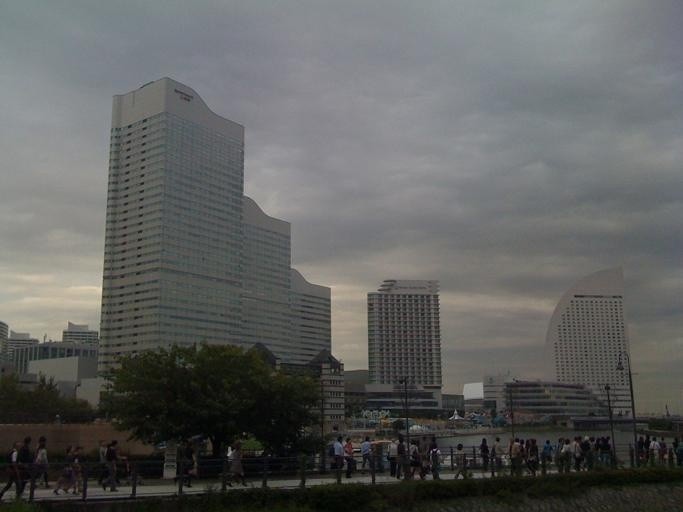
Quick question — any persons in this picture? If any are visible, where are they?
[261,448,270,456]
[97,440,127,493]
[53,445,86,495]
[628,434,681,469]
[541,435,617,476]
[334,435,354,482]
[454,443,468,479]
[226,440,248,487]
[479,438,539,477]
[1,432,51,502]
[360,434,442,481]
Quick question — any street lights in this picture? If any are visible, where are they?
[73,383,80,400]
[617,351,640,467]
[506,387,517,440]
[398,376,412,482]
[604,384,618,468]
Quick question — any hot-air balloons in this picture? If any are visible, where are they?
[399,424,455,440]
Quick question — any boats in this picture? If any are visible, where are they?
[195,423,319,471]
[341,430,396,452]
[165,453,194,471]
[454,424,505,436]
[32,448,164,478]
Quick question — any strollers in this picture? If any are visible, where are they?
[113,455,142,486]
